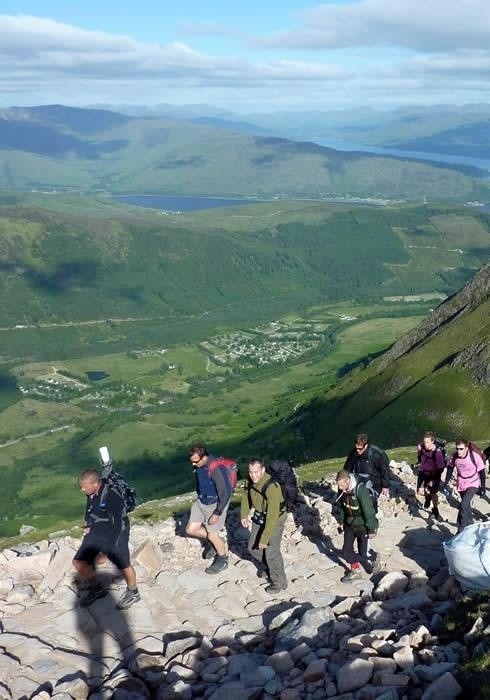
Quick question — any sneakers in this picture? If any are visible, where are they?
[425,495,432,508]
[205,545,217,558]
[205,556,228,574]
[80,582,105,606]
[261,570,269,578]
[372,552,381,575]
[266,585,286,593]
[432,507,438,515]
[341,568,362,583]
[116,588,140,610]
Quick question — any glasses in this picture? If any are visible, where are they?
[191,458,201,463]
[456,448,464,450]
[356,443,366,450]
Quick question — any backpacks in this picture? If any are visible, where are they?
[353,473,379,513]
[420,437,447,466]
[249,461,298,513]
[100,469,135,512]
[208,459,238,490]
[452,442,486,469]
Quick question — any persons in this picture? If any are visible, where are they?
[186,443,232,574]
[343,433,390,539]
[332,469,379,583]
[416,431,490,533]
[72,469,141,610]
[239,458,287,594]
